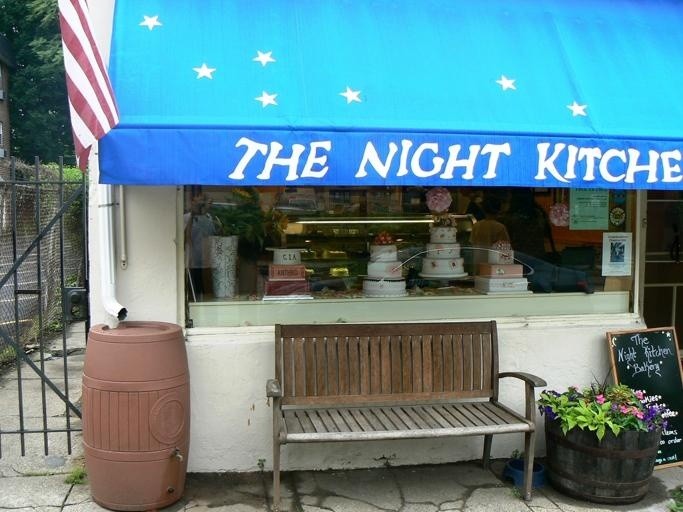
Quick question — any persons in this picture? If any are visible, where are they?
[182,191,223,300]
[457,186,559,276]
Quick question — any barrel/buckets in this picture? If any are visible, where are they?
[84,320,190,512]
[545,409,662,505]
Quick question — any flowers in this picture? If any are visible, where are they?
[533,364,669,441]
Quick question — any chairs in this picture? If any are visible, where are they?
[265,320,548,508]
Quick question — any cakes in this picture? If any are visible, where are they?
[333,268,348,276]
[422,227,464,274]
[474,240,529,292]
[362,231,407,294]
[330,267,334,274]
[265,249,310,296]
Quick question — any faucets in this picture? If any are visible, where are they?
[172,447,184,462]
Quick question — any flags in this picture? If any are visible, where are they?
[55,1,120,173]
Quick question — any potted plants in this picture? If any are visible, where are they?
[205,197,253,299]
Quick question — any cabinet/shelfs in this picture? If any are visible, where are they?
[260,214,478,289]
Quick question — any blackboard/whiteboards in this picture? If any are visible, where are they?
[607,325,683,472]
